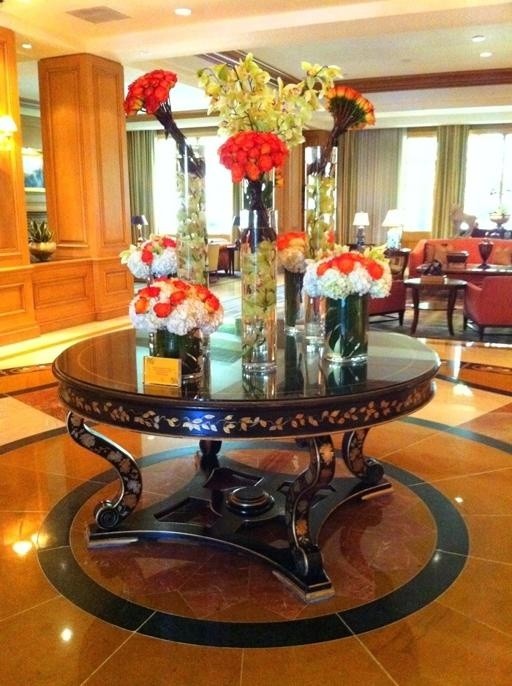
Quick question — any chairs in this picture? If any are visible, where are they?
[459,275,512,338]
[368,250,413,328]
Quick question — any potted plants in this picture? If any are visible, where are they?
[26,219,57,260]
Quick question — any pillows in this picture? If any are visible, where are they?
[422,241,452,268]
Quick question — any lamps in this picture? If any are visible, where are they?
[381,208,404,247]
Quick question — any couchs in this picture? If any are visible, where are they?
[409,238,512,280]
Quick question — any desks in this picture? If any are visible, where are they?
[51,320,443,605]
[404,276,467,337]
